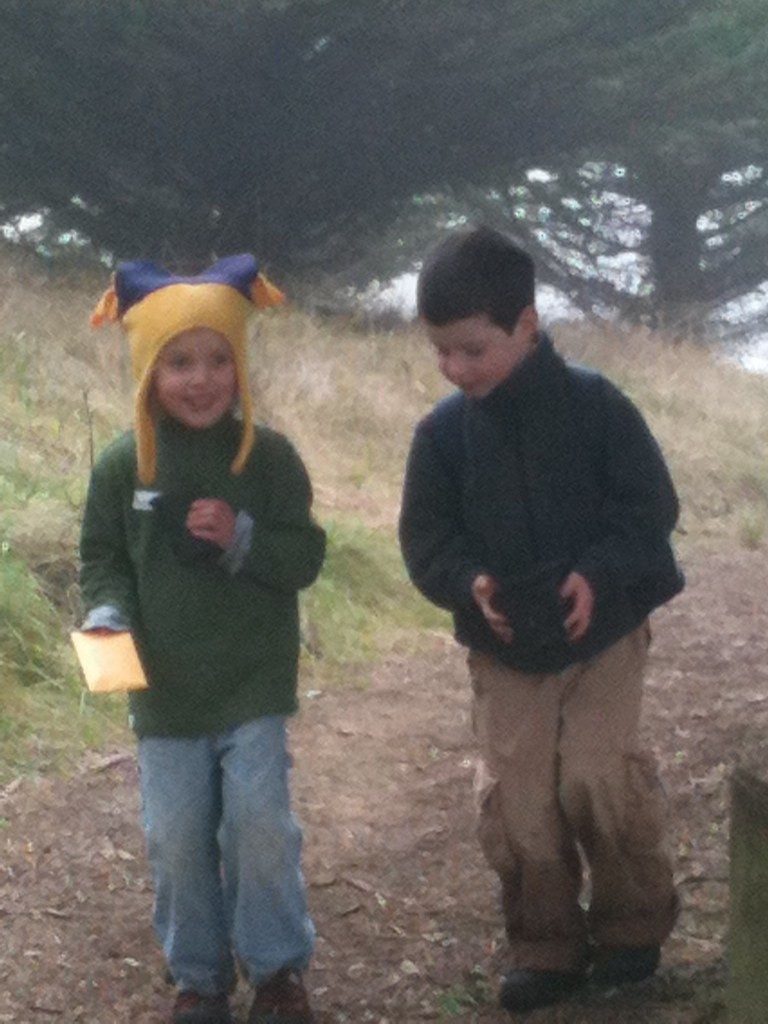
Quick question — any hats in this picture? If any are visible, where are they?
[91,253,287,485]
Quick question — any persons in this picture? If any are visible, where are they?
[70,252,328,1024]
[389,228,686,1011]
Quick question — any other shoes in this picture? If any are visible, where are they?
[171,967,310,1024]
[497,942,658,1010]
